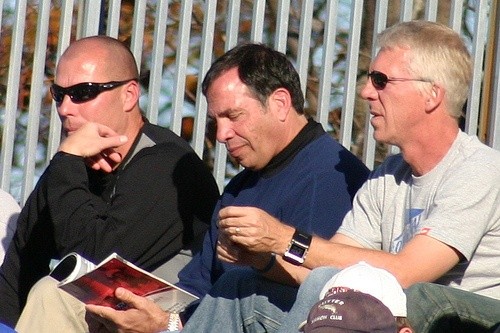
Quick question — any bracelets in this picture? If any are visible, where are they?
[249,254,277,274]
[166,311,181,333]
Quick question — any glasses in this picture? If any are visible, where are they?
[50,78,139,102]
[367,68,434,90]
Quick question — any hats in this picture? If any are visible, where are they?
[305,289,399,333]
[298,260,407,330]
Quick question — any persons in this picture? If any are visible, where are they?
[85,43,376,333]
[301,262,500,332]
[0,34,222,332]
[179,20,500,333]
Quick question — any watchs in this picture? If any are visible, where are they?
[282,228,313,264]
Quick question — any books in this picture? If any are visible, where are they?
[43,252,200,333]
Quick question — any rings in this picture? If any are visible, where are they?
[235,225,240,236]
